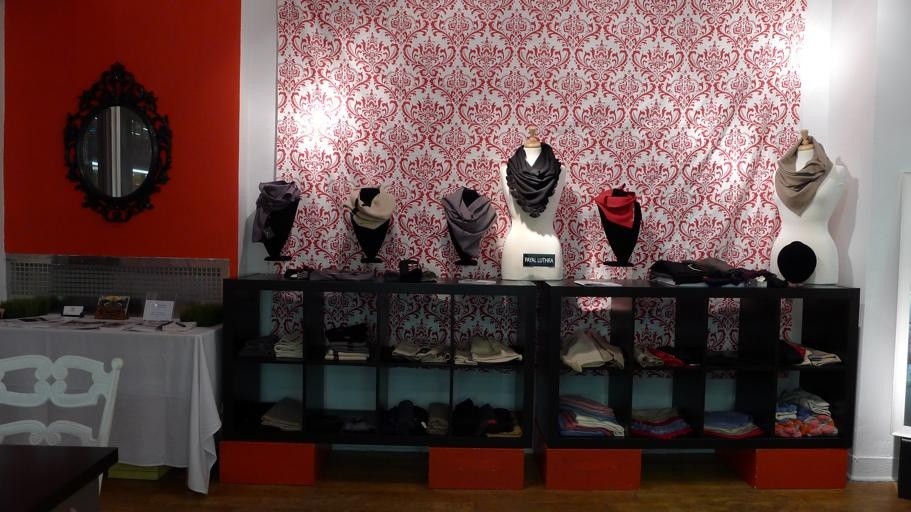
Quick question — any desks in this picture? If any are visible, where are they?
[0,306,224,481]
[0,443,118,512]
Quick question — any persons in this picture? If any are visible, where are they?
[500,126,565,282]
[771,128,846,286]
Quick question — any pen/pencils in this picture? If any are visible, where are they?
[175,321,186,328]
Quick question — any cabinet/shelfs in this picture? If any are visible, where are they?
[220,281,861,491]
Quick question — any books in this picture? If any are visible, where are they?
[0,317,198,332]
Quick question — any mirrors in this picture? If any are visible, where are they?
[60,61,172,224]
